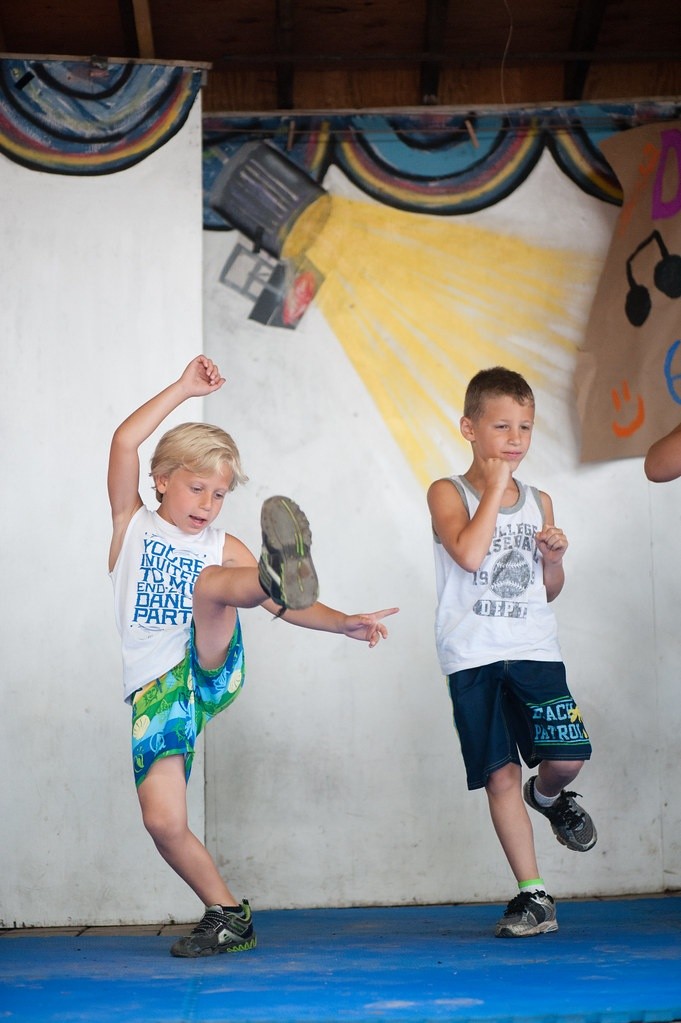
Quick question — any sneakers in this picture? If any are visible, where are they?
[256,495,320,612]
[495,888,558,937]
[522,774,599,853]
[170,898,255,957]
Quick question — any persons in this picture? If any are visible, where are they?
[426,364,599,942]
[100,352,400,957]
